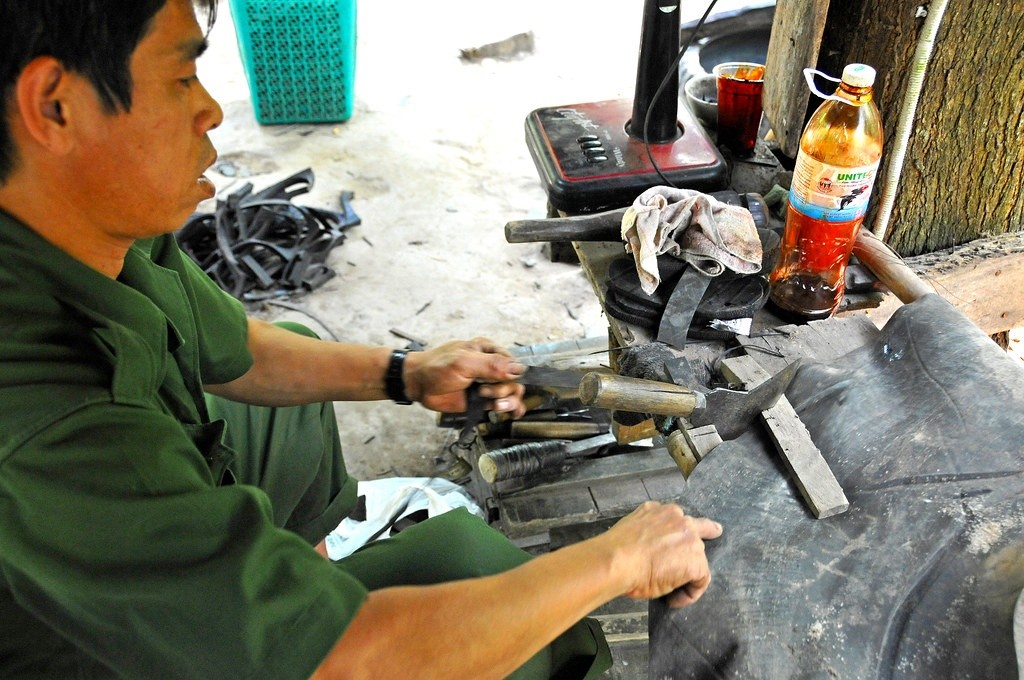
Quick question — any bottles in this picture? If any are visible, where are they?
[764,63,884,325]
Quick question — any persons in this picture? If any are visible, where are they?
[1,0,723,679]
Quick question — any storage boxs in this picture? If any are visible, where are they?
[228,0,360,123]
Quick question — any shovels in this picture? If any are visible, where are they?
[513,419,657,449]
[576,357,802,442]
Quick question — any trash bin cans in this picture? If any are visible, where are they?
[228,0,357,125]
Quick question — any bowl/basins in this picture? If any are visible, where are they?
[684,73,718,129]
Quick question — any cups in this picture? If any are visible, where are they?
[712,62,766,159]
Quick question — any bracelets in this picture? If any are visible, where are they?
[386,346,422,404]
